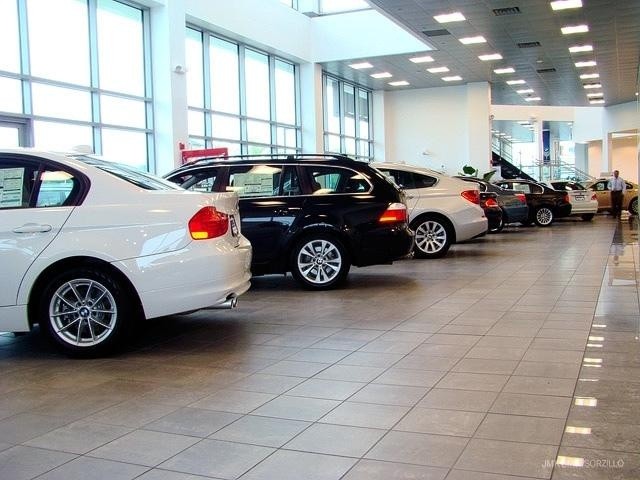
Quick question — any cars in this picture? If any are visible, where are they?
[0,147,640,358]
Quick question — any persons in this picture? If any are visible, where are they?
[607,170,626,219]
